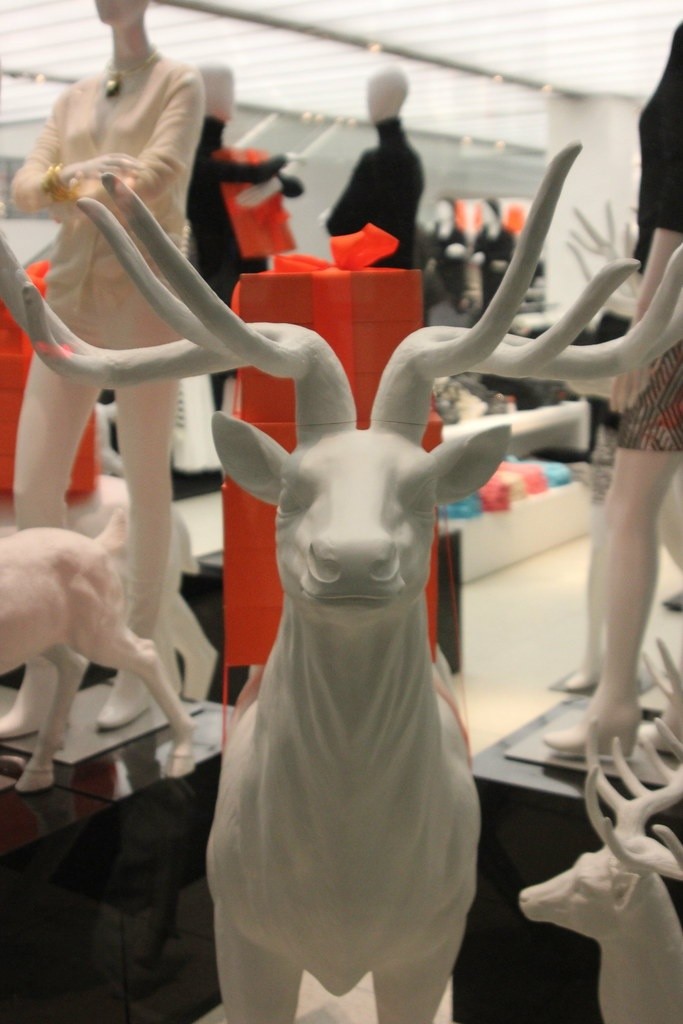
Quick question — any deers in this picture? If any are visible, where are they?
[2,139,683,1023]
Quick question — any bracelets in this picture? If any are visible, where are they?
[43,164,79,202]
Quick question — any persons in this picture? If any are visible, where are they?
[0,1,683,784]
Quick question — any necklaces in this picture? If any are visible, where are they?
[101,45,158,97]
